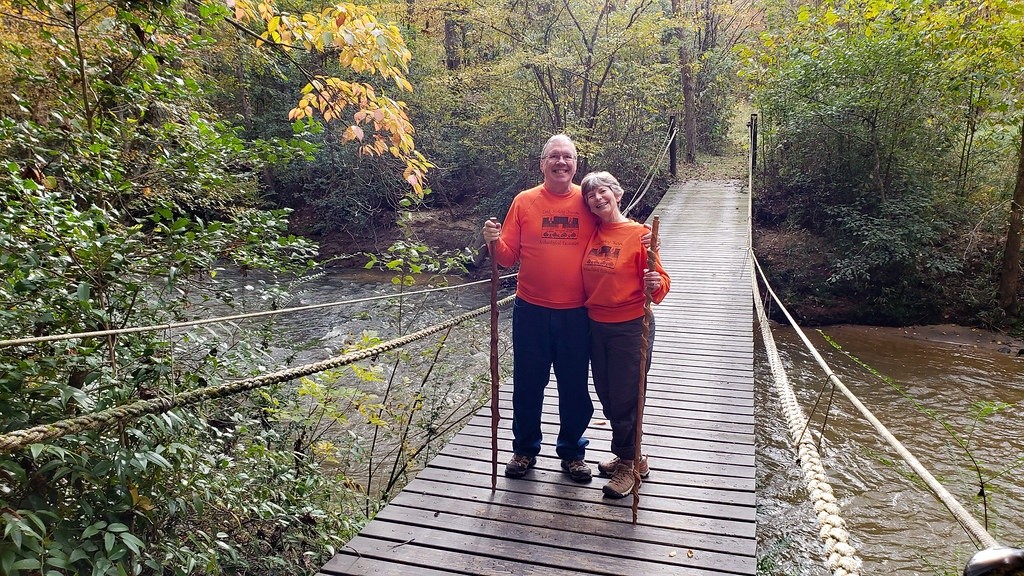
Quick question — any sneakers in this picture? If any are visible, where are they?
[505,450,536,478]
[602,461,642,498]
[598,450,650,478]
[561,457,592,481]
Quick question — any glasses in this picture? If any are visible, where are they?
[542,153,577,161]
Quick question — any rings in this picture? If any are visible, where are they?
[652,281,655,286]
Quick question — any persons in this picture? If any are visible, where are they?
[482,136,660,481]
[581,171,671,499]
